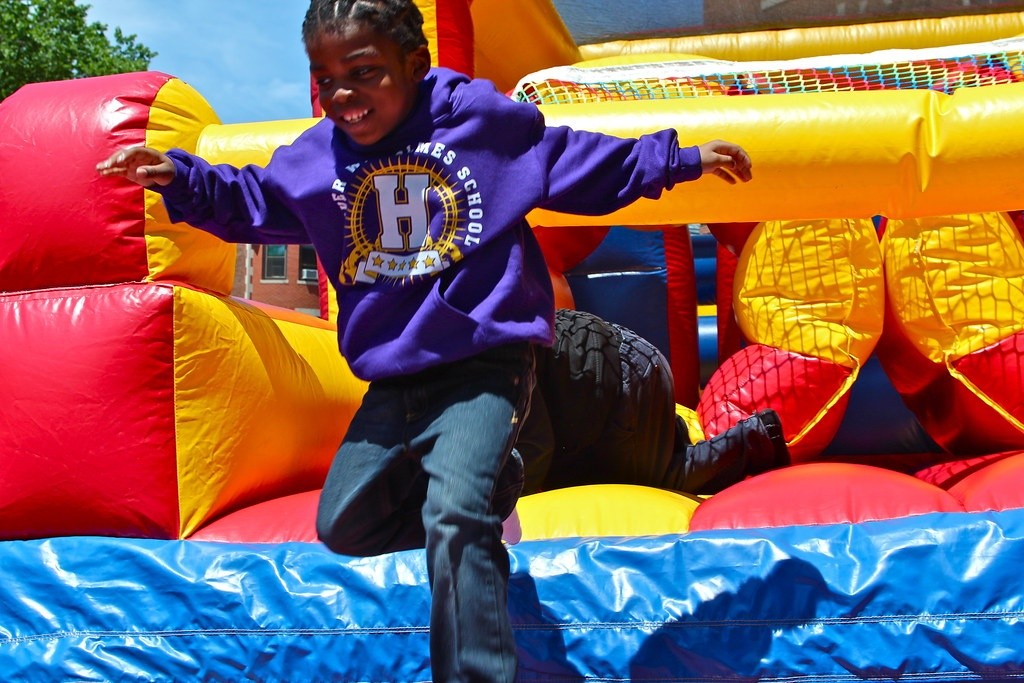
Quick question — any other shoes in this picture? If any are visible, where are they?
[493,447,524,522]
[759,409,791,467]
[676,415,693,445]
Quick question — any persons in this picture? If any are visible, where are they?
[516,310,790,497]
[95,0,755,682]
